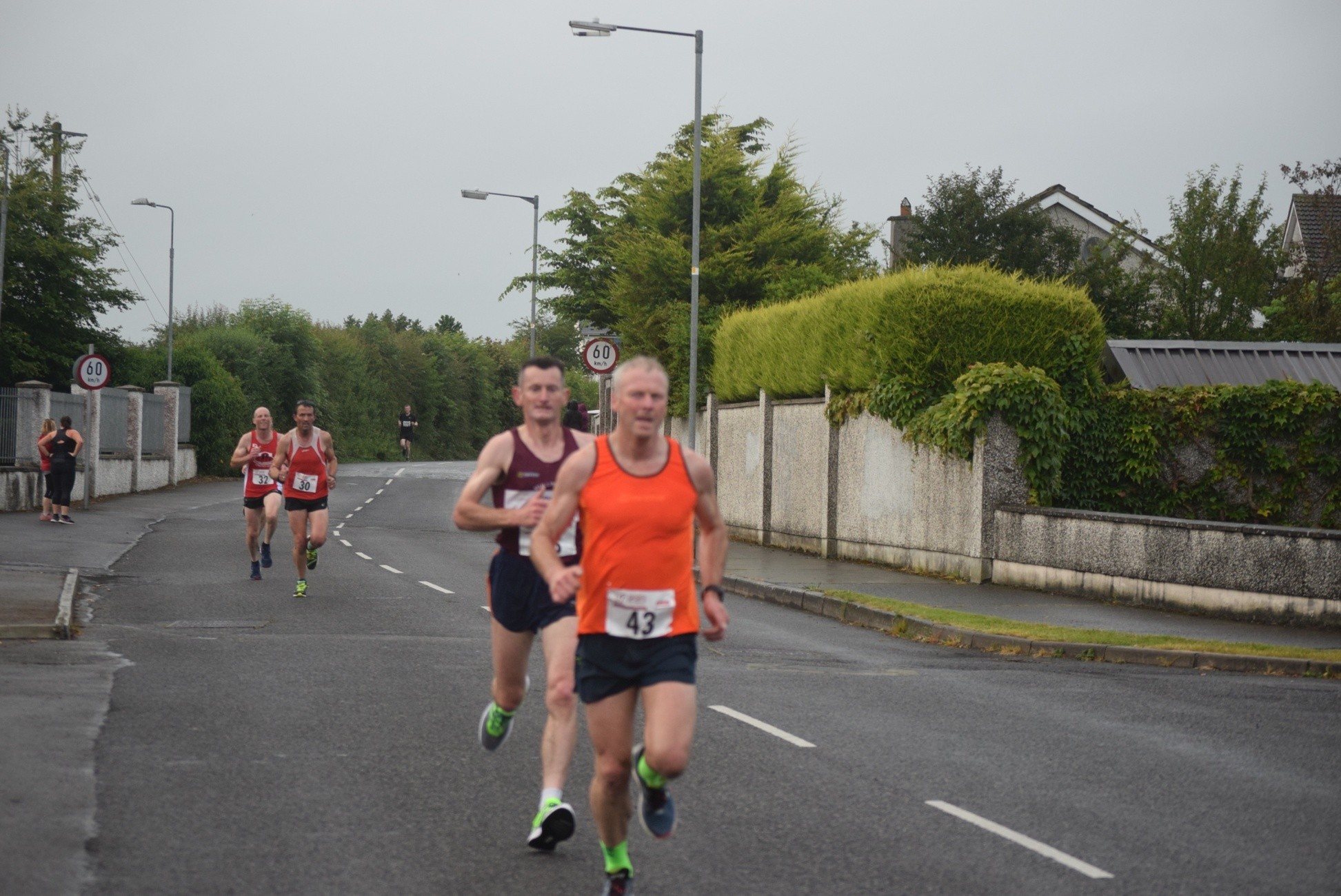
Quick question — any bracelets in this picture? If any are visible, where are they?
[699,584,725,603]
[276,472,285,485]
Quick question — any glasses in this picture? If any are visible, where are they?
[297,400,314,405]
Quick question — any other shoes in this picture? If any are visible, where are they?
[50,517,60,522]
[260,546,272,568]
[39,513,51,521]
[60,517,75,524]
[406,458,410,460]
[250,561,262,579]
[403,449,407,455]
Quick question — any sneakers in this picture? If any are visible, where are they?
[306,535,318,570]
[527,798,576,853]
[603,863,633,896]
[479,675,530,752]
[292,578,308,597]
[632,744,678,840]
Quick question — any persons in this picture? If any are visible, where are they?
[36,417,84,524]
[230,408,289,579]
[562,401,589,432]
[398,404,418,461]
[268,401,338,596]
[40,419,57,521]
[453,358,597,853]
[529,355,730,896]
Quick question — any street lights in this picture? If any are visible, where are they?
[567,18,705,450]
[131,199,176,380]
[459,189,539,356]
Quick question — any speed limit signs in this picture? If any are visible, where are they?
[78,354,110,390]
[583,337,622,374]
